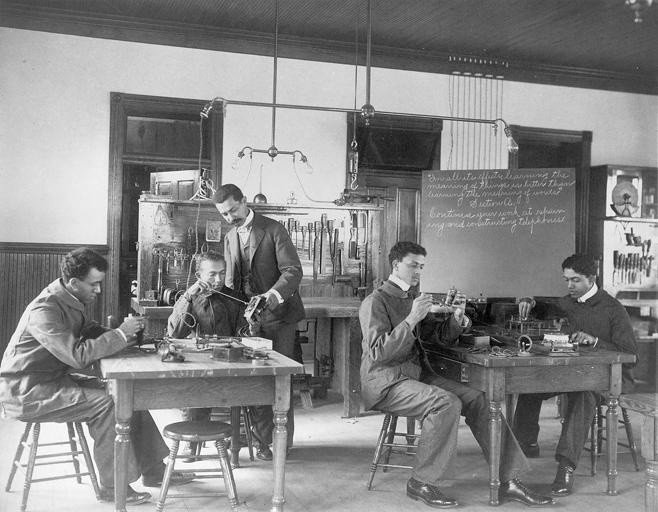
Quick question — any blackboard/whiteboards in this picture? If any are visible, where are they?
[417,166,577,299]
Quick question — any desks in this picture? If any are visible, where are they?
[425,340,641,509]
[616,391,658,511]
[104,341,307,512]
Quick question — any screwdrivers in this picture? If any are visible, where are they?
[202,282,250,307]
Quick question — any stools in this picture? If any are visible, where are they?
[581,397,642,480]
[2,414,104,512]
[194,405,256,462]
[152,418,244,512]
[363,407,430,495]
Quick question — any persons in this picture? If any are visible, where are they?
[512,254,639,498]
[253,194,267,204]
[0,248,198,505]
[358,240,554,509]
[167,250,273,462]
[213,183,307,457]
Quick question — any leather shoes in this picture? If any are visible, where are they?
[252,433,273,460]
[517,441,540,458]
[406,477,458,508]
[181,442,196,462]
[97,485,152,506]
[143,462,196,485]
[500,462,574,506]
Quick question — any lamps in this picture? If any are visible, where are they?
[228,1,315,174]
[194,1,521,158]
